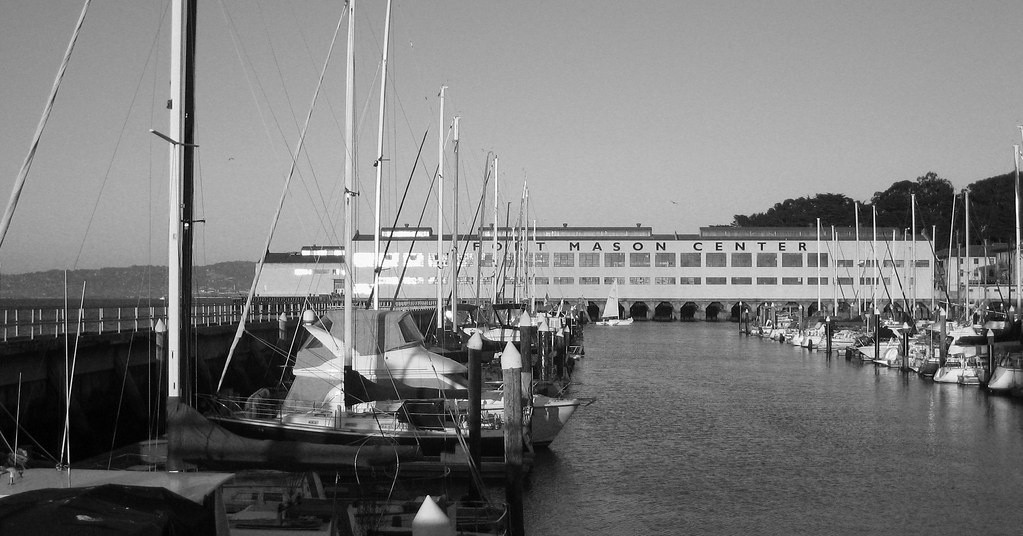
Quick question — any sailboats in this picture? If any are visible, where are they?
[0,0,597,536]
[595,278,634,327]
[738,144,1023,401]
